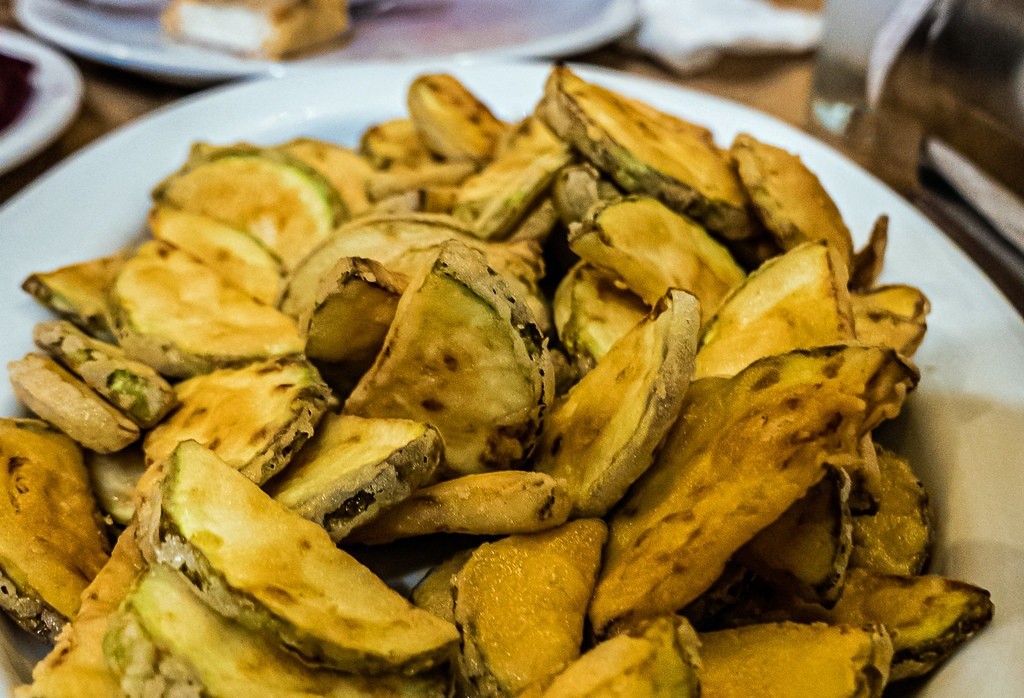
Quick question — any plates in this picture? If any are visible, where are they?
[1,46,1024,694]
[0,24,87,179]
[11,0,636,73]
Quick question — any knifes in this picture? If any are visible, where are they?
[928,137,1024,257]
